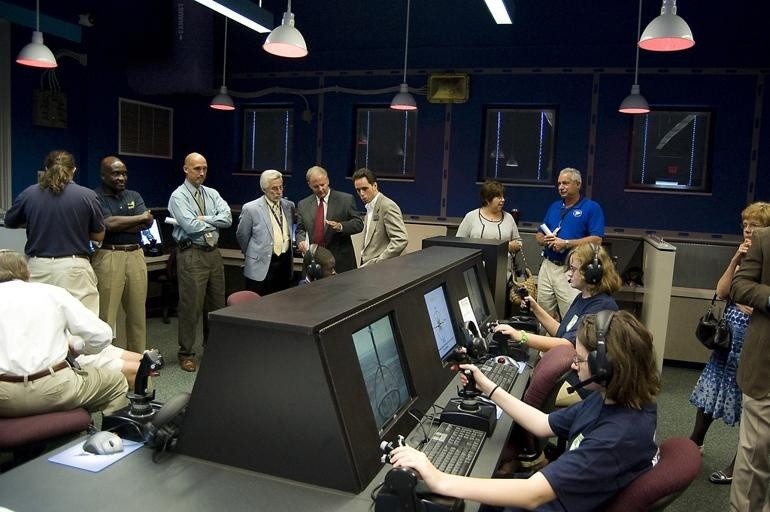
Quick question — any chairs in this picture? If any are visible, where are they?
[156,249,178,325]
[612,438,703,511]
[0,407,92,467]
[227,291,261,305]
[497,345,576,471]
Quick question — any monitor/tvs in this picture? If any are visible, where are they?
[424,284,460,363]
[353,311,415,435]
[292,222,301,242]
[140,217,162,247]
[463,265,491,326]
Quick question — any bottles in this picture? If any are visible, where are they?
[511,209,519,227]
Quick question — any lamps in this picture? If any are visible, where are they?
[485,0,512,27]
[618,1,650,116]
[638,0,696,52]
[208,18,235,110]
[263,0,308,59]
[198,0,272,35]
[391,1,421,111]
[17,1,59,70]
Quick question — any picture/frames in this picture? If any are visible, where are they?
[118,97,174,159]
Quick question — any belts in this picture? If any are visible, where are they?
[0,350,69,383]
[99,243,142,252]
[191,244,217,252]
[30,254,87,258]
[544,255,564,266]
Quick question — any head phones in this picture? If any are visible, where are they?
[462,320,489,357]
[588,309,617,387]
[582,241,604,286]
[142,390,192,451]
[307,244,322,278]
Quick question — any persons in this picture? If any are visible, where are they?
[353,168,408,267]
[299,246,335,285]
[168,152,232,373]
[0,249,149,417]
[390,311,664,510]
[689,202,769,484]
[90,157,160,377]
[236,169,297,297]
[455,180,522,320]
[536,168,605,334]
[4,151,105,318]
[493,241,622,353]
[729,226,770,511]
[296,166,364,274]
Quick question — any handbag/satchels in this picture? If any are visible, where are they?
[509,267,537,305]
[695,312,733,351]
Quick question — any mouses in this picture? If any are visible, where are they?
[493,355,520,369]
[82,430,124,454]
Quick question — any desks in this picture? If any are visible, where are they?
[358,349,540,512]
[0,432,376,512]
[144,247,305,274]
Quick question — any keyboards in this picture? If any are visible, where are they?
[420,421,487,477]
[473,359,519,420]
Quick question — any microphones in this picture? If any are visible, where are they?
[566,368,607,395]
[568,279,572,283]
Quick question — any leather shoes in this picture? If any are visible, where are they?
[710,470,733,485]
[180,359,196,372]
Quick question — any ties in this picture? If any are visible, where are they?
[196,190,205,216]
[312,199,324,244]
[272,205,282,256]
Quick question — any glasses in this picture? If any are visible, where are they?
[572,354,588,366]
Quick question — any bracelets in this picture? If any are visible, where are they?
[488,386,499,399]
[518,330,528,346]
